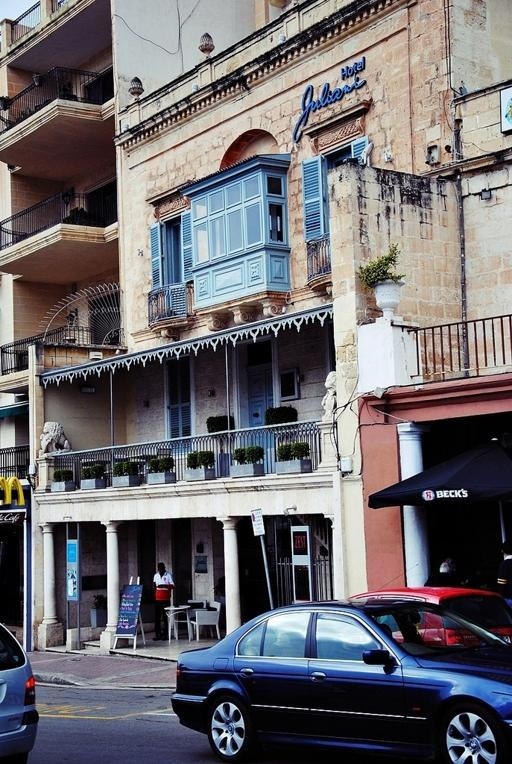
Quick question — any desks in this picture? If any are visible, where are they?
[164,604,191,644]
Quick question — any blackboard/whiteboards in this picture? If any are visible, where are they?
[114,584,143,636]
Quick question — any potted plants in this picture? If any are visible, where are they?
[275,442,312,474]
[148,457,175,484]
[112,463,140,487]
[229,446,265,478]
[360,242,407,319]
[185,451,215,480]
[80,465,105,489]
[51,470,75,491]
[90,594,107,628]
[205,415,234,476]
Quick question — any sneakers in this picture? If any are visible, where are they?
[152,636,168,641]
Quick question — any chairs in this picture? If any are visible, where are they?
[185,600,222,641]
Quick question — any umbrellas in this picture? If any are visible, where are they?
[366,428,512,545]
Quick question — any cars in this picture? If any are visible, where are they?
[0,623,39,763]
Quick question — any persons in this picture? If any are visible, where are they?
[493,540,511,626]
[435,556,474,589]
[151,562,175,642]
